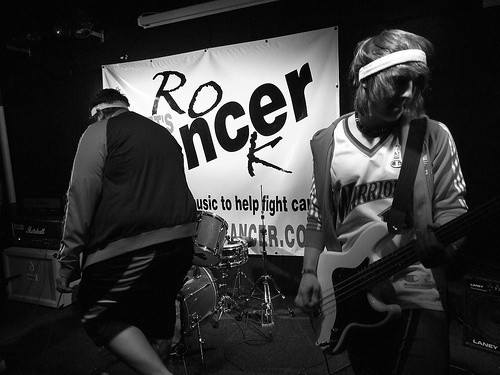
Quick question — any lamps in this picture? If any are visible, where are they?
[138,0,275,28]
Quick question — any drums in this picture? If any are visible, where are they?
[192,210,228,267]
[157,265,220,338]
[209,238,249,267]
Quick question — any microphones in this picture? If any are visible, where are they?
[6,274,20,281]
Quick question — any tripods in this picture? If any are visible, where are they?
[210,211,295,342]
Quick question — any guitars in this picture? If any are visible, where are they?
[305,195,500,355]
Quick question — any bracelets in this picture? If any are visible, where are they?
[301,267,315,276]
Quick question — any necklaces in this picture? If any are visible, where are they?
[354,111,398,139]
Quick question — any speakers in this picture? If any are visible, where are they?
[3,246,76,308]
[461,270,500,355]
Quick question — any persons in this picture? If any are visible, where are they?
[56,88,200,375]
[295,29,474,375]
